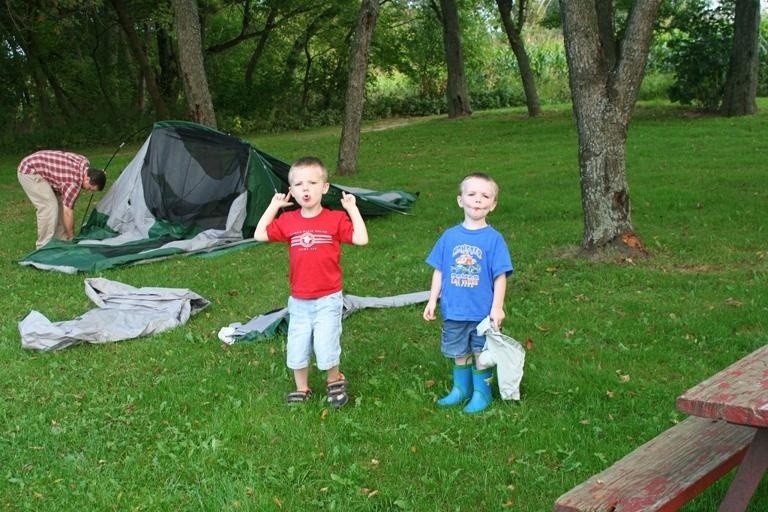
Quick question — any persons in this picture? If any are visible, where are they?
[423,173,514,414]
[17,150,106,249]
[254,156,368,409]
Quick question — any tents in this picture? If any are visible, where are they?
[18,120,419,276]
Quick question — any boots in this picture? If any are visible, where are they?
[437,365,472,405]
[462,365,495,413]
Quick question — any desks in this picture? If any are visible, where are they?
[675,344,768,511]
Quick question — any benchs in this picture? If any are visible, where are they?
[555,414,754,512]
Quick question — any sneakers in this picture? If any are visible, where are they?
[326,373,348,408]
[288,389,312,403]
